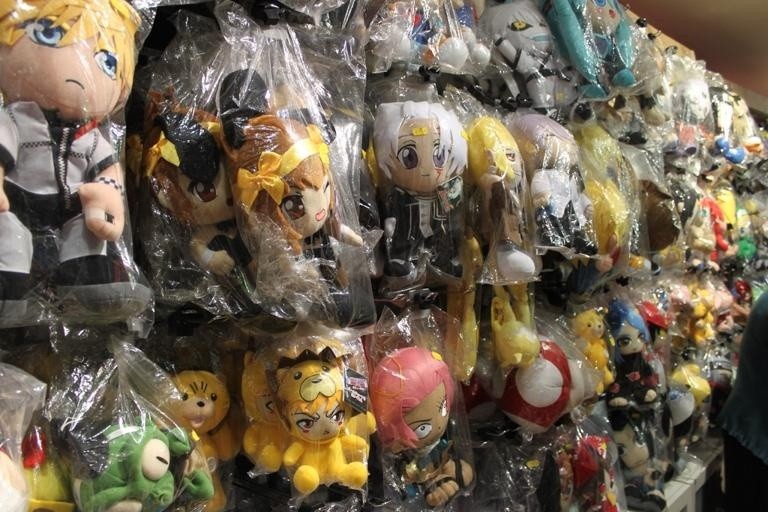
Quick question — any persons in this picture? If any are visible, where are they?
[0,0,768,511]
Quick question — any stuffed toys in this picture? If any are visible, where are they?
[1,0,768,512]
